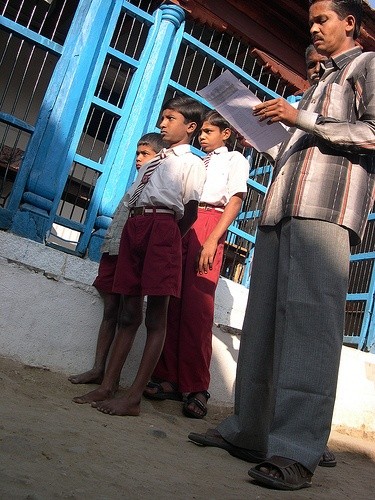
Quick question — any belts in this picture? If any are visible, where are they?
[128,207,175,217]
[198,204,225,213]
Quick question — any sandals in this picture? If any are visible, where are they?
[183,390,211,419]
[144,380,186,401]
[319,445,337,467]
[248,455,313,490]
[188,428,268,463]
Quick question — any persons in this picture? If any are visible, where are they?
[143,109,251,418]
[187,0,375,491]
[67,132,171,384]
[72,97,208,417]
[279,42,340,469]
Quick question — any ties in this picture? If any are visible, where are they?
[204,151,212,170]
[127,151,174,206]
